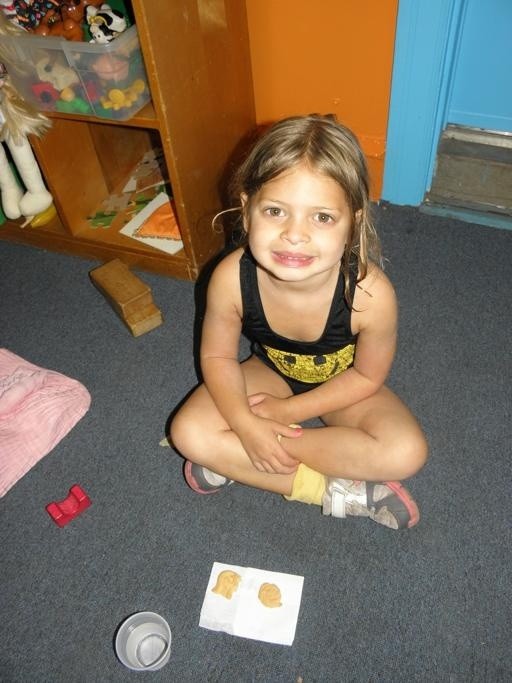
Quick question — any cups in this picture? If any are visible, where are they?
[115,611,172,671]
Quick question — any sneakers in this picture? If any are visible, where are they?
[321,474,421,531]
[184,459,235,497]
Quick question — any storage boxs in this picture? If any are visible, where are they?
[1,22,153,122]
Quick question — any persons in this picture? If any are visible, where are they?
[1,73,53,222]
[169,113,429,531]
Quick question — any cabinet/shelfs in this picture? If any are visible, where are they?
[0,0,256,281]
